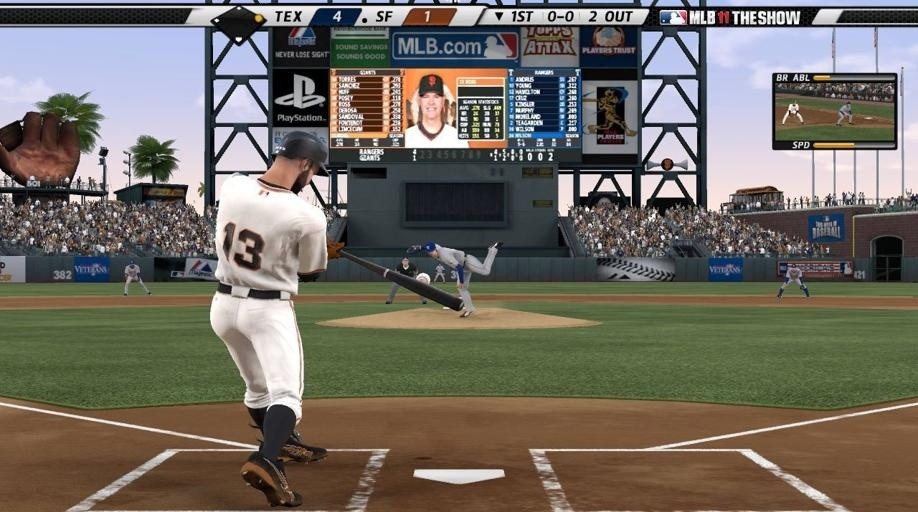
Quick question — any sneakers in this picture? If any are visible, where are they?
[239,451,304,507]
[249,423,328,463]
[460,309,474,319]
[385,301,392,305]
[488,241,505,249]
[422,301,427,305]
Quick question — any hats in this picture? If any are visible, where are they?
[129,259,135,266]
[418,75,445,97]
[270,131,331,178]
[424,241,436,251]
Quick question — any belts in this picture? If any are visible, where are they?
[216,282,281,299]
